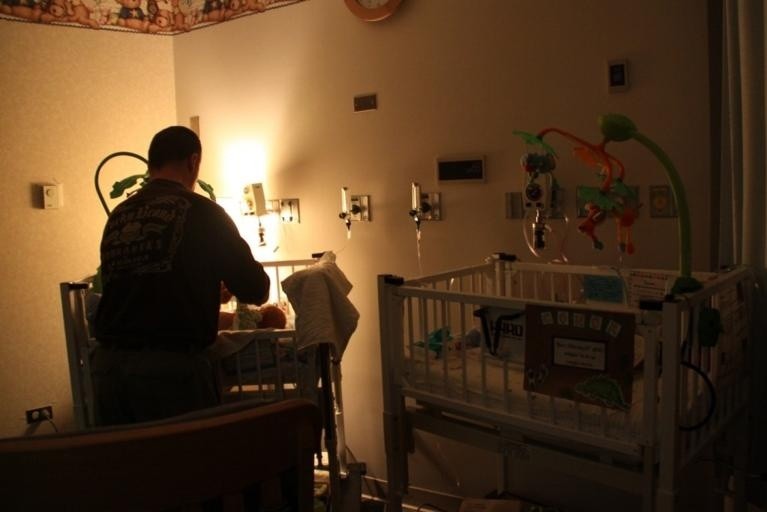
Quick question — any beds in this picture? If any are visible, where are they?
[58,252,346,510]
[374,253,758,510]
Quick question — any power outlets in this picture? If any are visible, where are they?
[25,405,52,424]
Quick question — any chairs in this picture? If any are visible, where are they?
[0,397,324,511]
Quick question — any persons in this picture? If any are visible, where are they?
[94,126,269,425]
[219,299,285,329]
